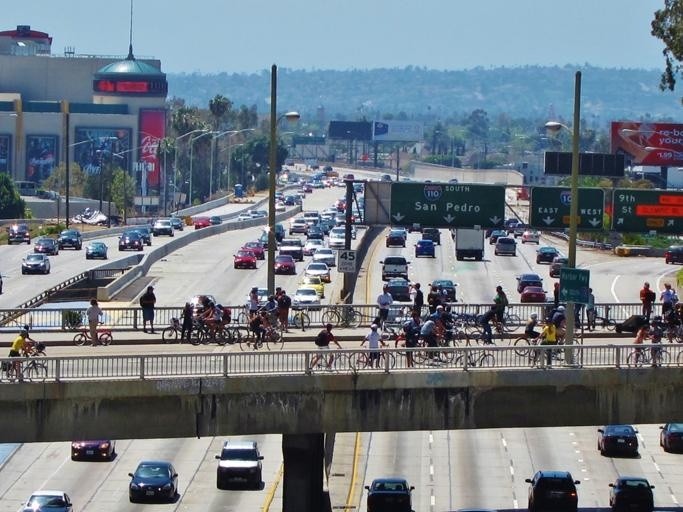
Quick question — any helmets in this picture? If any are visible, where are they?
[436,305,443,311]
[371,324,377,330]
[557,305,566,312]
[530,313,537,319]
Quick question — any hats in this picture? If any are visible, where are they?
[496,285,502,290]
[147,285,155,291]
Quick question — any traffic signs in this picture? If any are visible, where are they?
[528,180,604,237]
[607,188,682,238]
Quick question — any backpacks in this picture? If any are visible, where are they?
[139,293,148,307]
[315,330,330,346]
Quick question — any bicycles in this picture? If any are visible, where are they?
[66,319,116,347]
[0,357,50,383]
[14,342,51,365]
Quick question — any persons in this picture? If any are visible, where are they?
[480,305,499,344]
[310,324,342,370]
[403,282,454,368]
[22,324,34,357]
[181,285,292,350]
[525,284,594,369]
[140,285,156,334]
[377,284,394,322]
[87,299,103,346]
[493,286,508,324]
[633,282,682,368]
[361,323,384,369]
[10,332,30,378]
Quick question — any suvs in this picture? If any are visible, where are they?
[522,469,584,509]
[207,440,266,487]
[664,244,683,264]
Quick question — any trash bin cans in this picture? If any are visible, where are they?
[235,184,243,197]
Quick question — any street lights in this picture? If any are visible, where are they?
[429,122,566,181]
[0,113,18,118]
[543,120,584,363]
[267,111,309,308]
[615,124,682,188]
[49,126,261,224]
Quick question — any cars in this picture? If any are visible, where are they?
[593,424,642,460]
[363,477,417,511]
[655,422,682,453]
[127,455,179,503]
[66,440,118,463]
[17,489,74,512]
[607,478,657,509]
